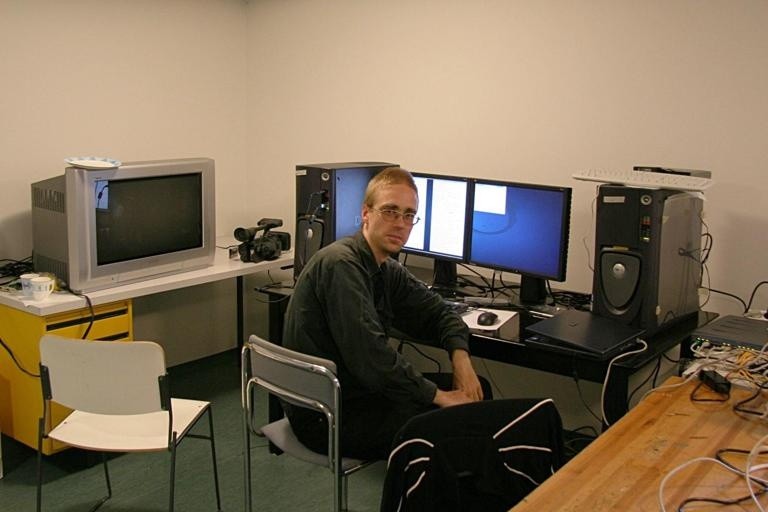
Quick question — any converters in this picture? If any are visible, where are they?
[701,371,731,393]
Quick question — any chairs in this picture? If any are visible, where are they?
[238,333,394,511]
[31,328,223,511]
[380,386,569,511]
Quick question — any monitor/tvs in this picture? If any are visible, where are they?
[31,157,216,295]
[466,178,572,309]
[400,172,471,291]
[94,180,113,215]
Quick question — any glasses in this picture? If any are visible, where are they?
[371,207,421,225]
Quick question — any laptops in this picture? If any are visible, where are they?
[523,309,649,362]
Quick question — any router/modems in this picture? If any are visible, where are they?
[691,314,768,352]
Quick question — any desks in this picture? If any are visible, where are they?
[0,231,300,472]
[253,261,720,457]
[505,344,767,512]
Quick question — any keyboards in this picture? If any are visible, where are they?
[571,166,715,191]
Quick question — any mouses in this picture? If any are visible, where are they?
[477,312,497,325]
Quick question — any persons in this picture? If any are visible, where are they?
[282,168,493,461]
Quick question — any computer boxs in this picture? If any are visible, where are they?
[593,184,703,332]
[294,161,400,286]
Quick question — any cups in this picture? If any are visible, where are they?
[20,273,39,296]
[30,276,55,302]
